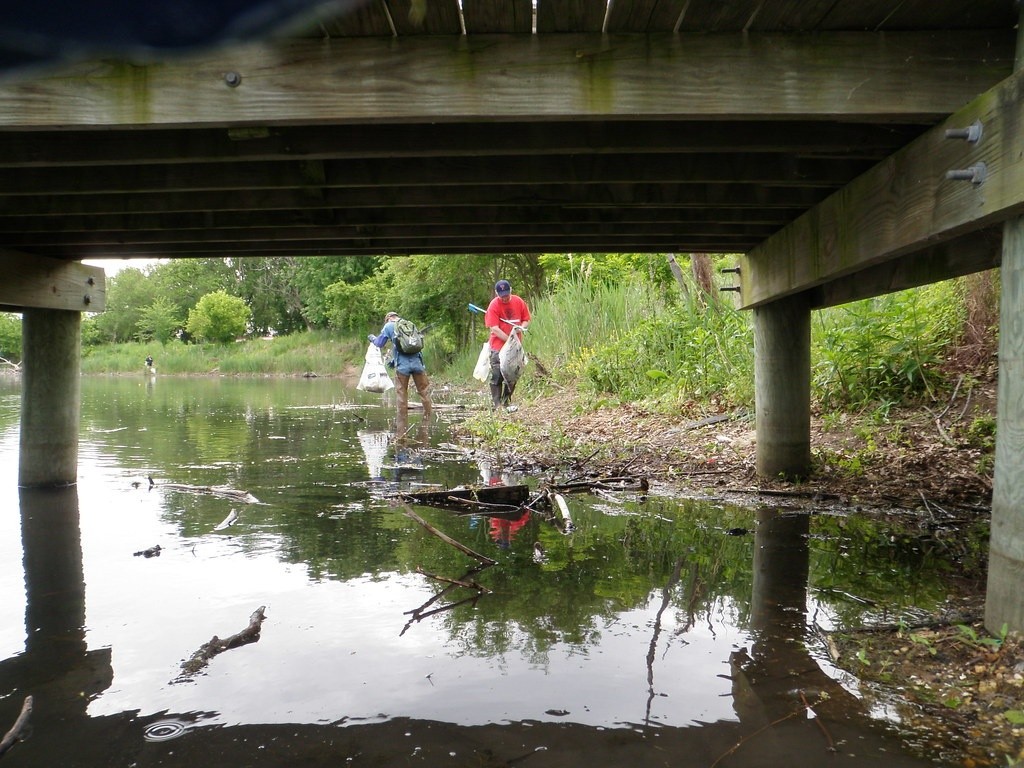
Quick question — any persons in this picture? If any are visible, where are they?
[144,353,153,369]
[485,279,531,414]
[386,417,430,509]
[367,311,433,418]
[487,461,532,549]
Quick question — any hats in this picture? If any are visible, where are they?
[496,541,509,549]
[385,312,398,323]
[495,280,510,296]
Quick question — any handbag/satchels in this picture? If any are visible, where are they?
[473,341,491,382]
[356,343,395,393]
[151,368,156,374]
[498,326,529,395]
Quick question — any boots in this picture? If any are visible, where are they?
[396,412,434,445]
[395,370,409,412]
[490,383,518,413]
[416,383,432,412]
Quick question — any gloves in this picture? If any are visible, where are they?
[388,359,394,370]
[367,334,377,342]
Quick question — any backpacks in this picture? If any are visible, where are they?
[392,319,424,356]
